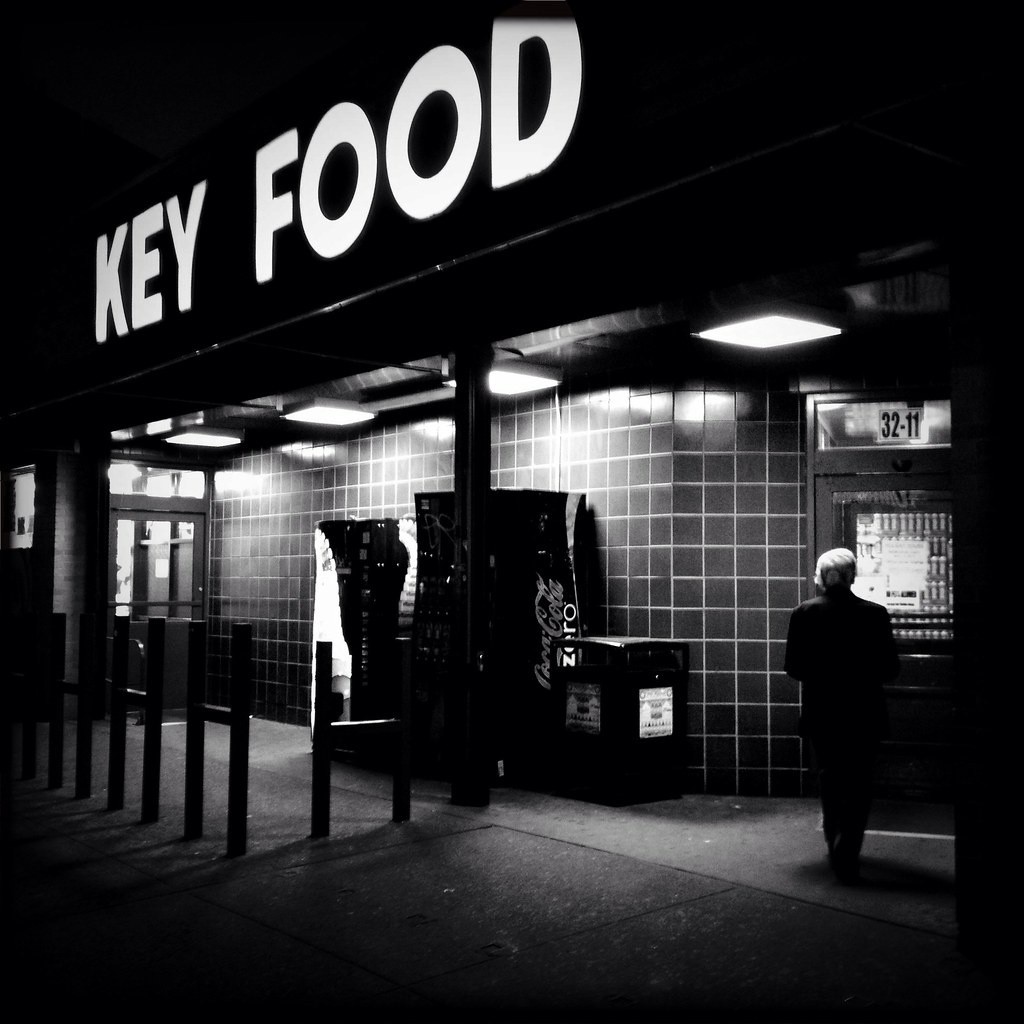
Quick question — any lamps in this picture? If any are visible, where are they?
[687,301,844,351]
[161,424,244,448]
[439,362,559,395]
[277,397,377,426]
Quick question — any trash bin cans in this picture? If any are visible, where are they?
[545,633,692,810]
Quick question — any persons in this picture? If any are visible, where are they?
[783,546,903,882]
